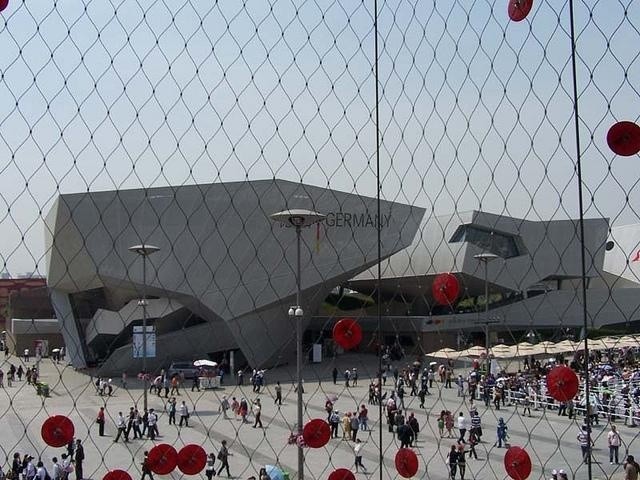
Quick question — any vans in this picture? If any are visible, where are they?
[168,361,209,379]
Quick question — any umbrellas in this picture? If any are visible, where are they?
[426,335,640,382]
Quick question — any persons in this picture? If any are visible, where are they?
[1,343,640,480]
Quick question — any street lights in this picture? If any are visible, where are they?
[126,241,162,420]
[474,250,501,375]
[270,204,328,479]
